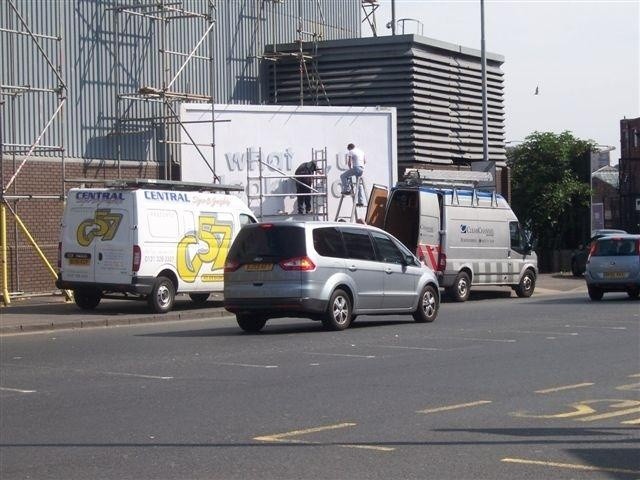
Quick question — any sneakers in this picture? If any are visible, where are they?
[298,210,311,214]
[356,204,363,206]
[341,191,351,194]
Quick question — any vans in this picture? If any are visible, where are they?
[222,220,442,330]
[55,176,264,315]
[383,182,539,302]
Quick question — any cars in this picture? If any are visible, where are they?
[584,233,640,301]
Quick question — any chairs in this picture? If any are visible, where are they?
[605,240,640,255]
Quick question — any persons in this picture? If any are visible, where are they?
[293,160,324,215]
[340,142,367,207]
